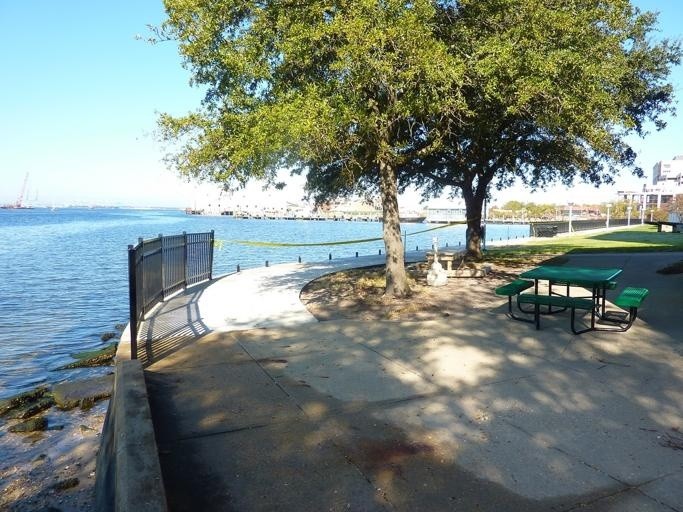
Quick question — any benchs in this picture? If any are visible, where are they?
[496,280,534,316]
[615,287,649,331]
[551,281,619,313]
[517,294,594,335]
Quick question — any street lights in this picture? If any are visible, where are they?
[627,207,632,225]
[650,210,655,222]
[606,204,612,227]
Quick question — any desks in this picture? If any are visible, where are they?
[647,220,683,233]
[427,246,465,270]
[520,266,622,328]
